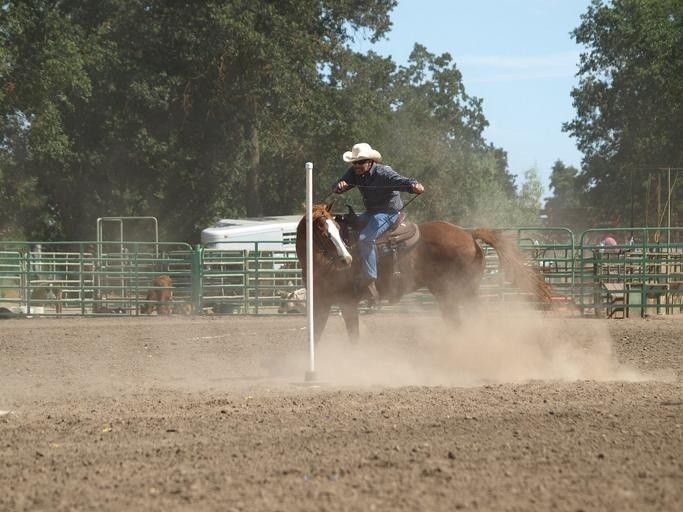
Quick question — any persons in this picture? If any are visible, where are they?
[22,245,42,296]
[331,142,424,300]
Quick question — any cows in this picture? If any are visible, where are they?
[277,286,306,315]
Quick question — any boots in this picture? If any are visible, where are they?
[359,279,376,307]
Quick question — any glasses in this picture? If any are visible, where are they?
[352,160,369,164]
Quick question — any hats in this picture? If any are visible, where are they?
[343,143,381,162]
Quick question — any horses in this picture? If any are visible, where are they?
[295,200,556,349]
